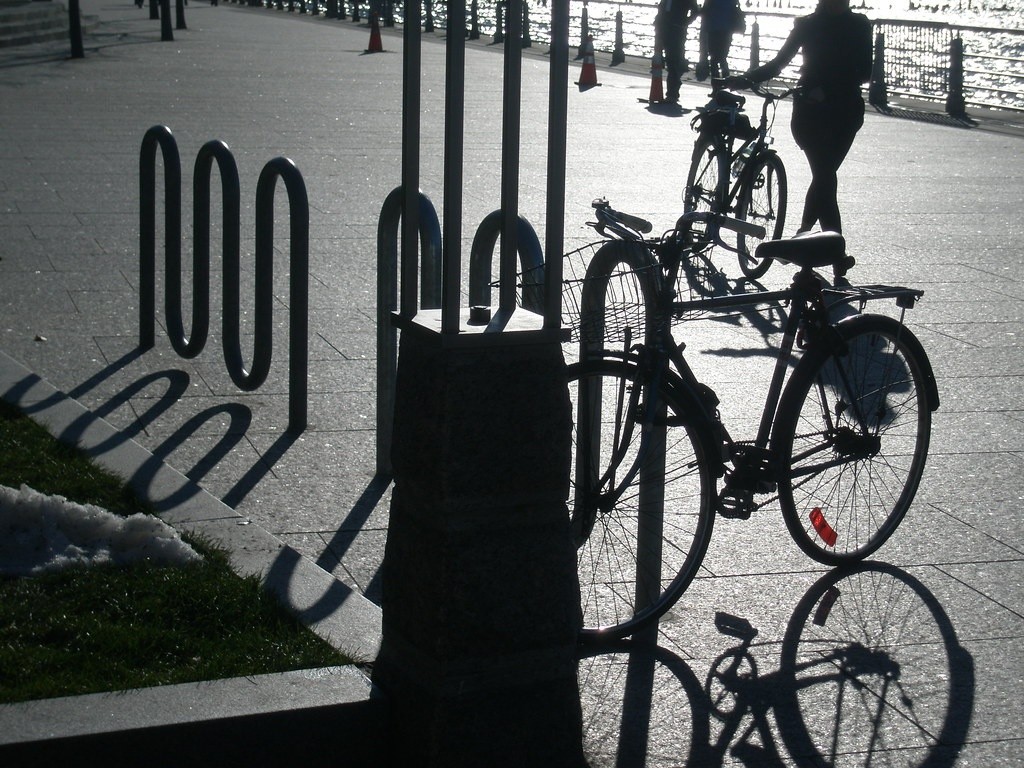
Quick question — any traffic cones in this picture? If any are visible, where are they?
[574,35,603,87]
[363,11,387,53]
[637,46,666,105]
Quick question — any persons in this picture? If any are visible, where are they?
[700,0,739,97]
[655,0,698,102]
[711,0,873,276]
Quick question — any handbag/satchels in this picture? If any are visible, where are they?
[735,3,746,34]
[695,58,709,82]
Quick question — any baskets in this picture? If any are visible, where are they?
[488,239,716,344]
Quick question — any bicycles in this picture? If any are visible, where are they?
[681,78,811,282]
[489,195,941,644]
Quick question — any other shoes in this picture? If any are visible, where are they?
[663,95,678,103]
[833,255,855,276]
[708,90,715,98]
